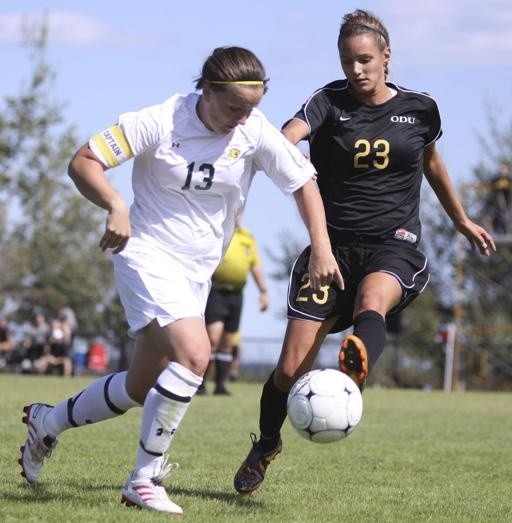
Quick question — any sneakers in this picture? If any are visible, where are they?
[120,476,184,514]
[17,402,59,484]
[233,432,282,493]
[339,333,369,395]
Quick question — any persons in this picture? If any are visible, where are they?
[194,208,269,396]
[236,12,496,497]
[0,307,78,377]
[19,44,348,514]
[230,331,240,380]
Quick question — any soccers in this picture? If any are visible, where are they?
[287,369,363,443]
